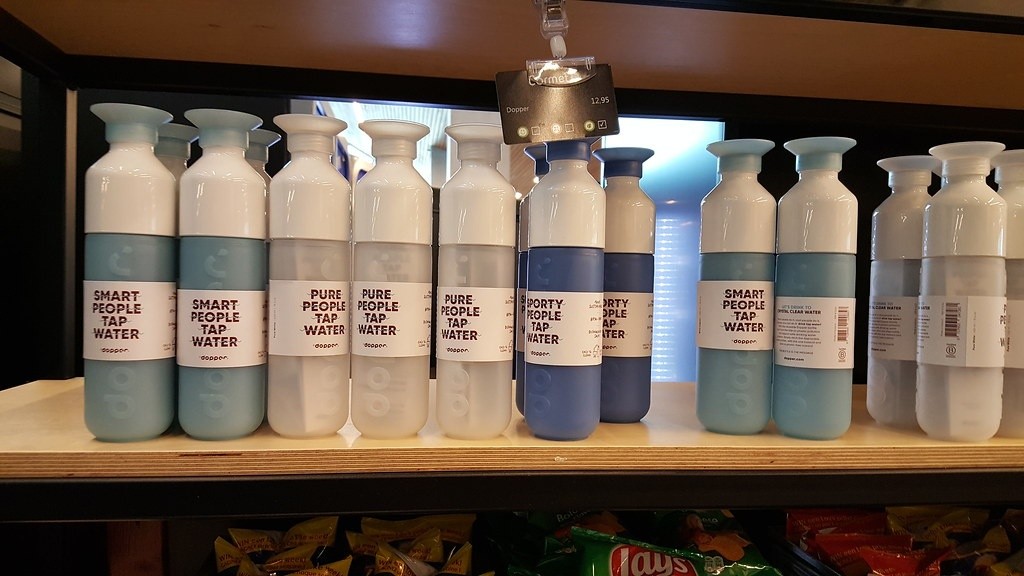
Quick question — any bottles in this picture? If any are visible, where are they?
[915,139,1009,445]
[989,149,1024,443]
[245,126,284,183]
[433,121,518,446]
[264,107,353,442]
[590,145,658,426]
[520,145,550,185]
[82,103,171,445]
[695,137,778,438]
[864,155,940,432]
[771,136,860,444]
[523,137,602,443]
[353,118,434,442]
[175,108,268,443]
[153,122,200,179]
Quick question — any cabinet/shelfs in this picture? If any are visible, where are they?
[0,2,1024,518]
